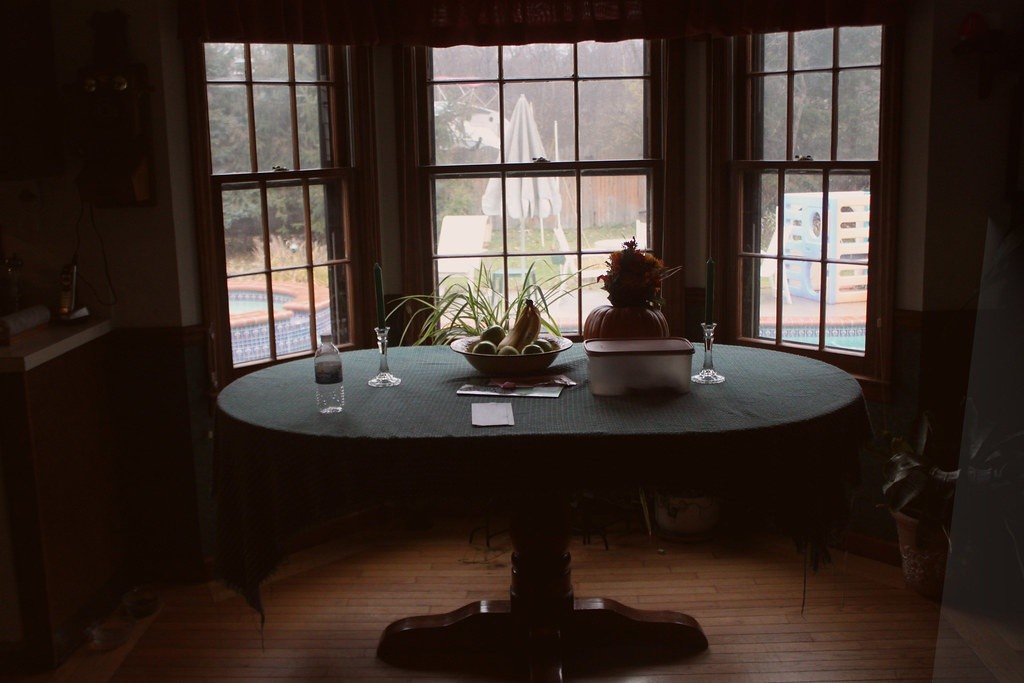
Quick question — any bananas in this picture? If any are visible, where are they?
[498,300,541,352]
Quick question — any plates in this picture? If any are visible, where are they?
[449,333,574,375]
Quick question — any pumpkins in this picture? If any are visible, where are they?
[584,305,670,341]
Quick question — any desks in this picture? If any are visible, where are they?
[219,348,862,682]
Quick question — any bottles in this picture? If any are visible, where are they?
[0,256,24,316]
[313,333,344,415]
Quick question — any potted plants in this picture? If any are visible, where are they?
[861,400,964,605]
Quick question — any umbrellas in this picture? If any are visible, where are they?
[482,96,562,275]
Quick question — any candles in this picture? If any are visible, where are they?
[375,263,386,328]
[704,258,717,328]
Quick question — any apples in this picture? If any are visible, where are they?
[472,325,552,356]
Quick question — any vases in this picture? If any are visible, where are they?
[653,496,721,543]
